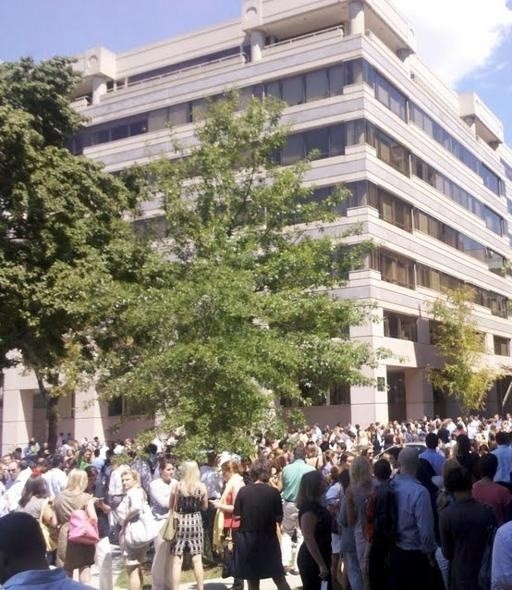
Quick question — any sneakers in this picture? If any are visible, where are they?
[284,566,299,575]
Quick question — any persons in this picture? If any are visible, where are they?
[233,414,511,589]
[1,433,246,590]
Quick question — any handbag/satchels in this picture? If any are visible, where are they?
[361,486,397,550]
[222,547,233,577]
[69,510,98,545]
[110,528,119,545]
[125,518,157,549]
[163,513,178,541]
[41,521,59,553]
[477,525,501,589]
